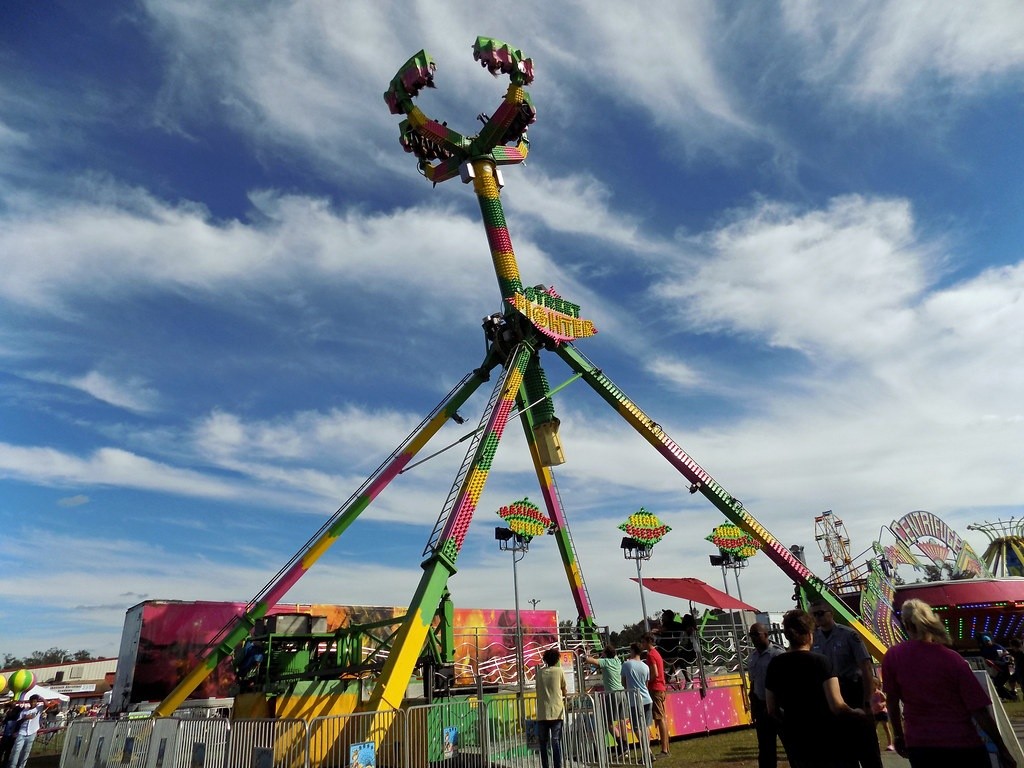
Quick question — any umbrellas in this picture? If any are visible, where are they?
[629,577,753,632]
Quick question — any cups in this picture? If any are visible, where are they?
[997,650,1003,655]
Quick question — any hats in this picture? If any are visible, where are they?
[982,636,991,641]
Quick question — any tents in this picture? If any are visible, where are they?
[8,685,70,722]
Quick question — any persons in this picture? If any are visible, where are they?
[981,634,1024,698]
[881,597,1017,768]
[534,649,568,768]
[67,703,107,718]
[0,694,61,768]
[585,631,671,764]
[747,598,894,768]
[680,613,699,690]
[653,609,682,682]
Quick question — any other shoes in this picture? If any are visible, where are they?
[656,751,671,757]
[886,745,894,750]
[614,743,630,759]
[638,753,656,764]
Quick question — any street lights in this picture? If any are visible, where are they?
[493,525,532,732]
[709,554,751,710]
[619,535,650,631]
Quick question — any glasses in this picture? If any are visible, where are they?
[813,610,832,618]
[749,631,764,637]
